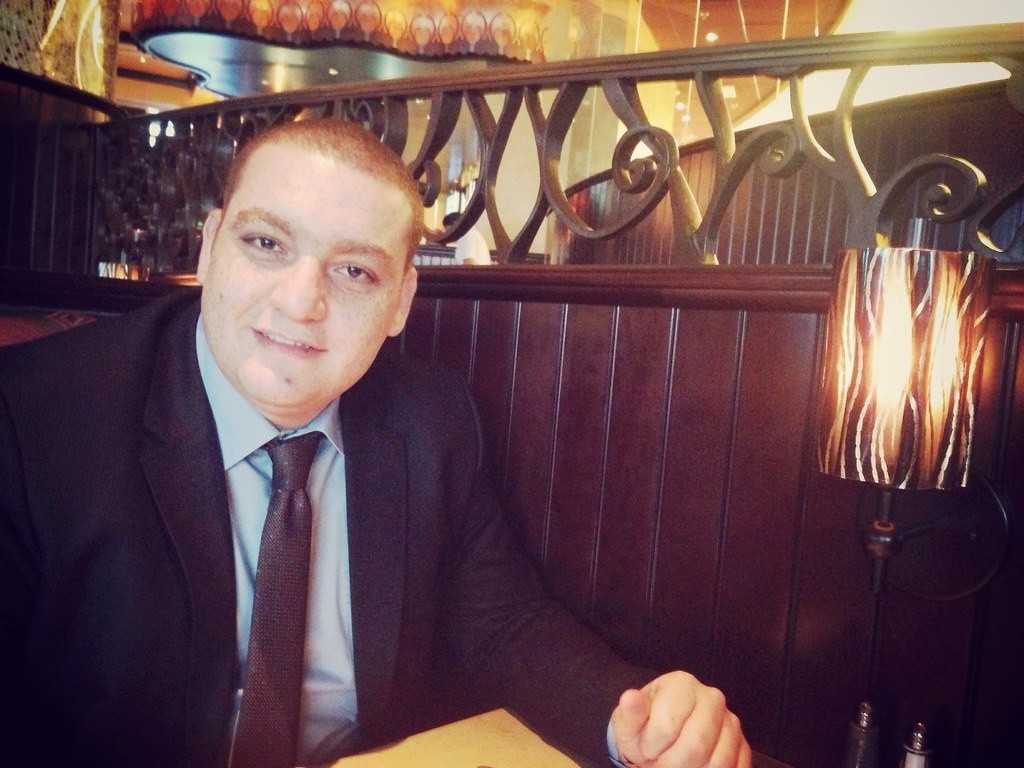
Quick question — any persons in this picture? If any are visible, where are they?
[443,213,491,265]
[0,120,752,768]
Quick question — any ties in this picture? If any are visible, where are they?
[233,431,321,768]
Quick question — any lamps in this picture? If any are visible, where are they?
[815,246,999,767]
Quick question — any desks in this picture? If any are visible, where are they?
[321,709,794,768]
